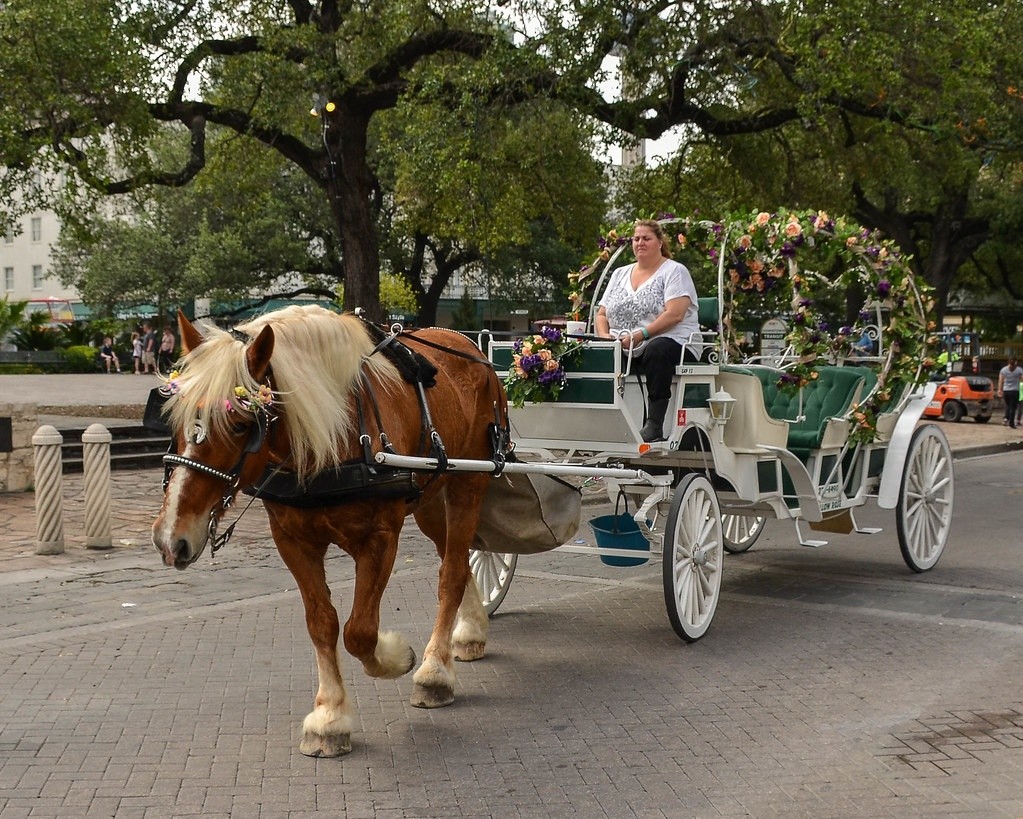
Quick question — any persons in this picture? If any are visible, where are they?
[158,328,175,373]
[833,333,846,350]
[100,338,121,374]
[855,333,873,352]
[131,332,144,374]
[141,321,157,374]
[596,219,703,442]
[997,355,1023,429]
[920,320,947,380]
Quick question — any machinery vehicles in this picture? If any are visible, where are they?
[922,332,993,424]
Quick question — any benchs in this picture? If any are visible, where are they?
[720,364,913,510]
[98,347,136,374]
[0,351,69,374]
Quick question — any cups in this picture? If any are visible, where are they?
[567,321,587,343]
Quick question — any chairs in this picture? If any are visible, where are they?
[594,297,719,443]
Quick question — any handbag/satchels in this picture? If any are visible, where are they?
[157,347,162,354]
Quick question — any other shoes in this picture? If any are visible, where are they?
[1009,423,1016,429]
[1015,421,1022,426]
[1001,420,1008,425]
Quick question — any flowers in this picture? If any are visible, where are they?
[501,328,584,409]
[163,370,274,412]
[565,203,944,445]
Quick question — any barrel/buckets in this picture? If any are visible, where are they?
[587,490,654,568]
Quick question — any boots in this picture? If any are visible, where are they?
[638,396,670,442]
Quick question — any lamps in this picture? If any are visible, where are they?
[707,384,737,444]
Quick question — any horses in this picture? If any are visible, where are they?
[149,306,511,758]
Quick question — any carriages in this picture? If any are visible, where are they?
[151,207,956,758]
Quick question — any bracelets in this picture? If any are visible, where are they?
[640,327,649,340]
